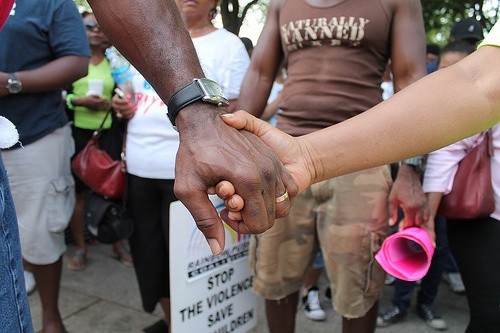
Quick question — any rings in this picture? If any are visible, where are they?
[276,189,288,203]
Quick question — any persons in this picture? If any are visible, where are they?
[207,16,500,223]
[111,1,250,333]
[375,210,447,329]
[427,44,440,63]
[0,0,300,333]
[0,0,91,333]
[233,0,430,333]
[61,12,126,271]
[306,252,330,321]
[421,123,499,333]
[437,41,475,71]
[449,18,483,48]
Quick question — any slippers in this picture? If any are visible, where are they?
[66,244,88,271]
[110,248,135,269]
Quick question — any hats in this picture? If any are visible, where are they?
[449,19,485,42]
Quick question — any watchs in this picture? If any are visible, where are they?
[7,71,23,95]
[167,78,230,132]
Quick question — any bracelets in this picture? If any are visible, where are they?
[66,94,78,110]
[402,157,427,173]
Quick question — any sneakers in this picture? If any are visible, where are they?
[376,301,448,331]
[442,269,466,293]
[301,285,328,321]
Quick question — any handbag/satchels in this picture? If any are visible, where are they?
[70,101,128,200]
[435,127,495,219]
[83,188,134,244]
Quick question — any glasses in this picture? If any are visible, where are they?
[84,21,101,29]
